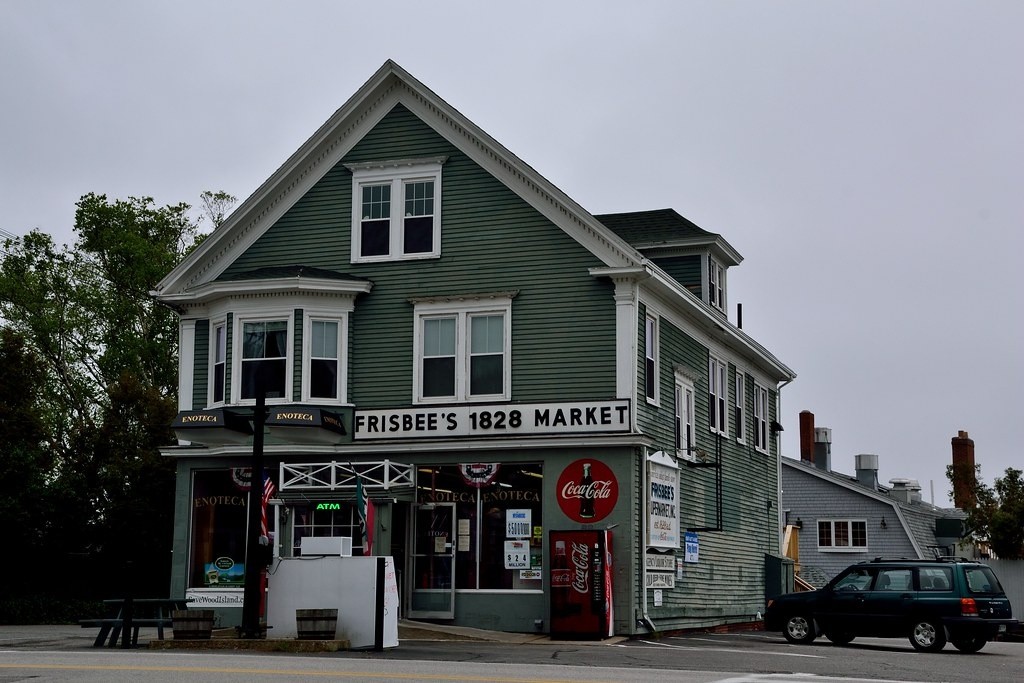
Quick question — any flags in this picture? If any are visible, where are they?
[357,479,374,556]
[259,466,276,546]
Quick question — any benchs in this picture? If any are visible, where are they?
[79,618,174,649]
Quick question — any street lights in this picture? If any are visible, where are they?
[221,372,282,638]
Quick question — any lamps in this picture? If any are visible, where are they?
[771,420,784,434]
[795,516,803,530]
[880,515,887,529]
[662,446,705,457]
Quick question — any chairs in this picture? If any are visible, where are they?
[876,575,891,590]
[920,576,945,590]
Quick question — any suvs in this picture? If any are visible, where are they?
[764,554,1020,654]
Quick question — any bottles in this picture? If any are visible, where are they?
[551,541,571,617]
[578,463,595,518]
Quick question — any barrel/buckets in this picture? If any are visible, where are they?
[172,609,214,639]
[295,608,338,639]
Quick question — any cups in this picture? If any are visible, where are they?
[207,571,219,583]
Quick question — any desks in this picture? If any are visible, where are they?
[91,598,196,649]
